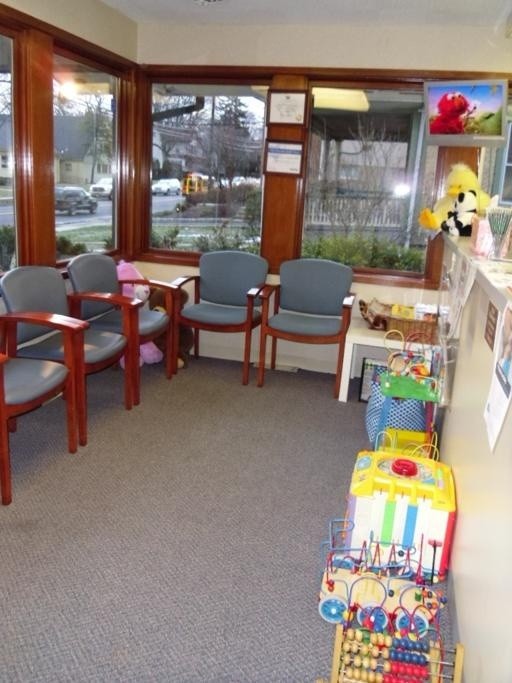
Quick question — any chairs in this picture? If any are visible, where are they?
[1,265,140,446]
[257,259,355,398]
[0,312,82,505]
[67,253,177,406]
[170,251,269,386]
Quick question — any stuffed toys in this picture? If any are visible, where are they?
[418,162,491,229]
[117,258,164,370]
[441,190,478,237]
[468,106,503,136]
[429,91,470,134]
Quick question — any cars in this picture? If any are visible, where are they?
[151,179,181,196]
[89,178,113,201]
[231,176,245,186]
[55,186,98,216]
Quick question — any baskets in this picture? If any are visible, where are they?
[371,297,441,343]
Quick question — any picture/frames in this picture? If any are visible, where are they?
[266,89,309,127]
[262,138,305,177]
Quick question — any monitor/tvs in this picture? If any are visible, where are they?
[422,79,507,147]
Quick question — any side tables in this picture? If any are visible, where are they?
[340,315,436,403]
